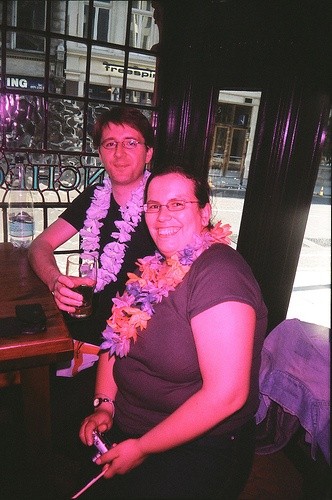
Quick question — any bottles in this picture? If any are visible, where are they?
[8,156,33,248]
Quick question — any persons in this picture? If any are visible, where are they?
[79,164,269,500]
[26,105,212,346]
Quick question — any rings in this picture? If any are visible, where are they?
[51,291,58,297]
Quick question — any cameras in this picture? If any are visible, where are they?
[90,430,113,463]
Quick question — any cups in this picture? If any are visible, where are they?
[67,253,97,318]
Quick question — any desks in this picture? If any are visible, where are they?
[0,250,74,491]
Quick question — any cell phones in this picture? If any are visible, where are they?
[15,304,46,334]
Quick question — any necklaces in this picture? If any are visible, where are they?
[101,232,213,358]
[80,170,151,293]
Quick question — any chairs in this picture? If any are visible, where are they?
[240,318,332,500]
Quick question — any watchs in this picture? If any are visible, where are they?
[92,398,115,407]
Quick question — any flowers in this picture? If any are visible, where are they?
[79,171,152,292]
[100,220,234,360]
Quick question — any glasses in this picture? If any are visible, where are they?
[139,198,200,214]
[100,138,145,149]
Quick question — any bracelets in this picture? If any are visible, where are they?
[94,393,114,418]
